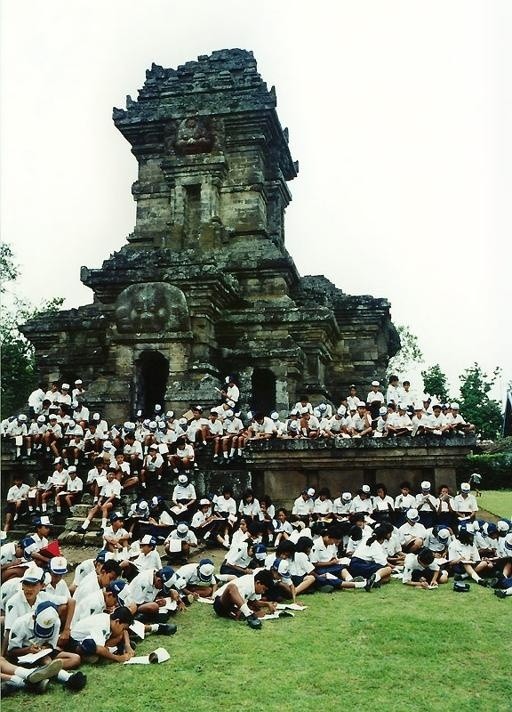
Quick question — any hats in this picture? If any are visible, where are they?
[157,566,177,594]
[177,522,189,538]
[96,550,114,564]
[1,376,238,513]
[50,556,69,574]
[31,516,54,527]
[33,601,59,639]
[273,557,292,578]
[197,559,215,582]
[138,533,156,544]
[244,399,463,421]
[372,380,382,388]
[20,565,45,585]
[252,543,268,560]
[106,579,130,608]
[301,481,512,558]
[110,511,124,522]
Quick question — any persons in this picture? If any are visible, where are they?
[1,375,512,693]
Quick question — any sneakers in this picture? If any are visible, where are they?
[64,671,87,693]
[183,594,193,606]
[74,526,89,533]
[95,527,105,537]
[348,570,512,593]
[26,658,63,683]
[246,613,262,629]
[155,622,177,635]
[24,679,50,695]
[0,530,7,541]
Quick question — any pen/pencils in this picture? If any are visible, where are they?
[29,640,41,650]
[194,592,200,599]
[457,551,463,558]
[123,649,125,655]
